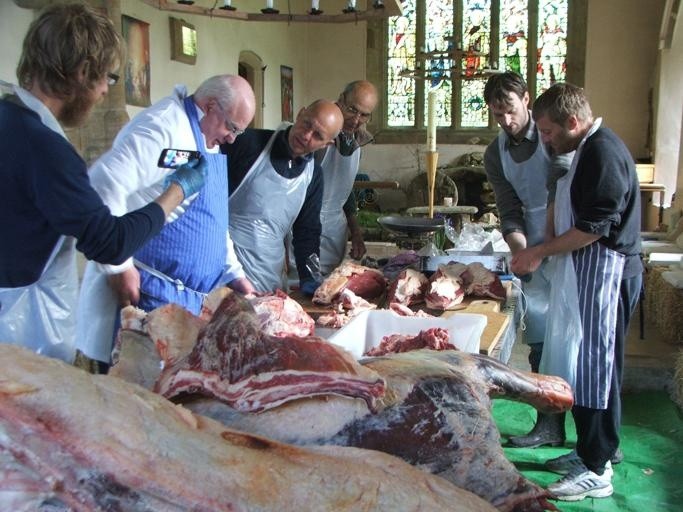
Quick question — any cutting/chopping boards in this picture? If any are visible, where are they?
[449,279,513,311]
[440,299,510,354]
[287,283,388,319]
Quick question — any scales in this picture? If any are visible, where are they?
[376,214,445,251]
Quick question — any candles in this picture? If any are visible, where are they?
[427,90,437,151]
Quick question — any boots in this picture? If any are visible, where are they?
[508,354,565,449]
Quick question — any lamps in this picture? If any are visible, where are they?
[143,0,403,22]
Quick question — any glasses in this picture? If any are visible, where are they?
[210,97,244,135]
[343,92,372,123]
[107,72,120,86]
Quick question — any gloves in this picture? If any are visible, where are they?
[349,235,366,261]
[168,155,209,200]
[301,280,320,294]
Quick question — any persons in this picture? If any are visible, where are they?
[284,81,378,292]
[218,100,343,298]
[511,83,644,500]
[73,75,253,376]
[484,72,575,449]
[0,3,209,365]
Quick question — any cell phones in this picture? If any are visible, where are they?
[157,148,200,169]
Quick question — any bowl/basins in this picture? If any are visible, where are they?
[322,308,488,361]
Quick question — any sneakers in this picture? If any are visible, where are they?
[546,466,612,501]
[544,450,625,476]
